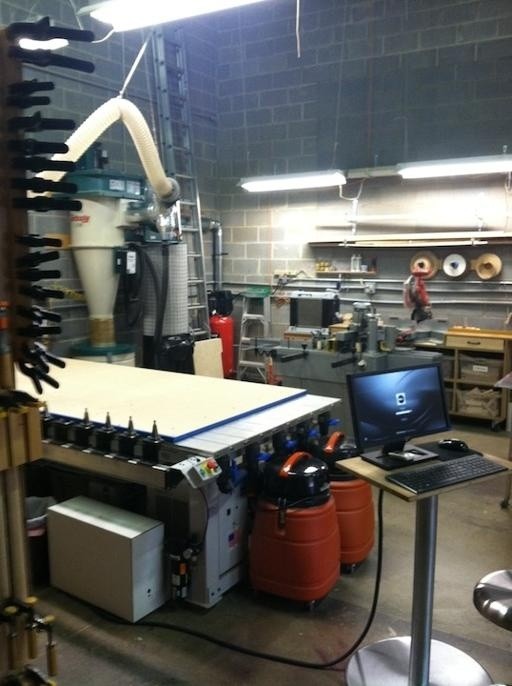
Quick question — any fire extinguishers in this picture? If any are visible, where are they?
[210,309,236,378]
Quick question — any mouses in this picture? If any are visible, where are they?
[438,437,470,452]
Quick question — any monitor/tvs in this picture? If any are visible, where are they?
[345,361,452,471]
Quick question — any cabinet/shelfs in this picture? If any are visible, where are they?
[410,326,512,431]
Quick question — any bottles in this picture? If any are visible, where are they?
[350,253,362,271]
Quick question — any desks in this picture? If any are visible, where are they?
[333,437,512,684]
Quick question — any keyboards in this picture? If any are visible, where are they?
[385,454,507,495]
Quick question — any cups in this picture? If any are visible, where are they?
[360,265,368,272]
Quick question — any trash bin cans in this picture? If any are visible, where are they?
[23,496,57,587]
